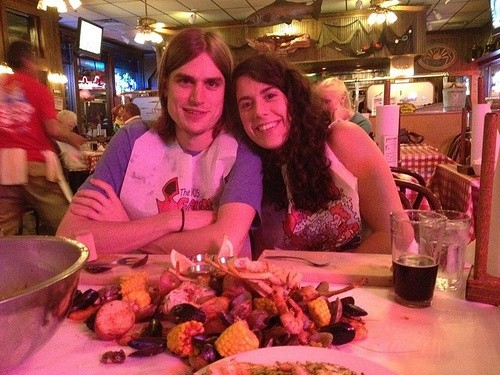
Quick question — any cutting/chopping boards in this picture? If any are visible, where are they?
[256,249,392,288]
[75,253,173,286]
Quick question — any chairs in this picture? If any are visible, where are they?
[391,166,444,223]
[448,130,471,165]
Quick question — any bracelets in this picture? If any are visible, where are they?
[179,209,184,232]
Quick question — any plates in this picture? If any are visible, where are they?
[192,345,398,375]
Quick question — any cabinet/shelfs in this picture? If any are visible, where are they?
[68,45,107,139]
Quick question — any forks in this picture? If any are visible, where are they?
[265,255,333,267]
[84,256,138,266]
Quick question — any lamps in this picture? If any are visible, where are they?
[36,0,81,13]
[368,0,400,25]
[133,1,167,44]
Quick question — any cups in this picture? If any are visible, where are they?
[417,210,472,294]
[388,209,448,309]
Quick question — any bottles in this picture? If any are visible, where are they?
[96,124,101,136]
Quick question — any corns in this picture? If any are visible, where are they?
[121,270,151,310]
[253,296,279,315]
[166,320,204,356]
[215,320,259,357]
[308,298,331,326]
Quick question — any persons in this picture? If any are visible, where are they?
[55,110,77,157]
[121,103,141,123]
[87,119,98,137]
[316,78,372,133]
[233,55,420,254]
[0,39,85,237]
[55,28,262,260]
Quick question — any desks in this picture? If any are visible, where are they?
[400,143,449,206]
[436,164,483,215]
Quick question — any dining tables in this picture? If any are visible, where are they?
[27,283,499,375]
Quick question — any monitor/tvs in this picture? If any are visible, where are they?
[75,17,103,61]
[489,0,500,38]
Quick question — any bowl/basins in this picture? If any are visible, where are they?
[0,234,91,371]
